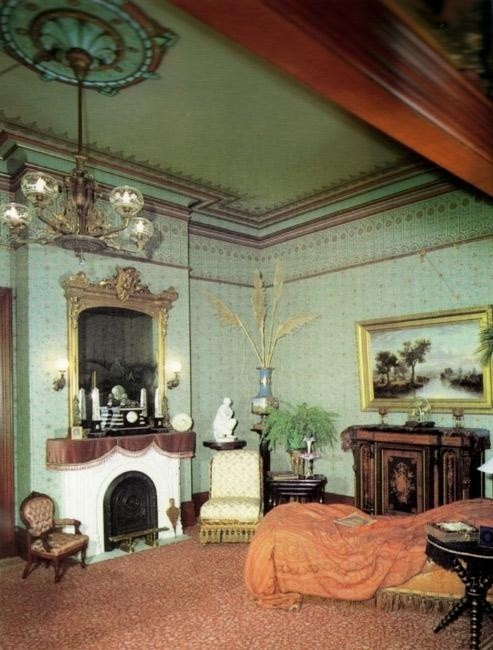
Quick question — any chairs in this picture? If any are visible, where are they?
[200,451,266,546]
[15,490,90,582]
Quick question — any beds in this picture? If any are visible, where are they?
[240,496,492,613]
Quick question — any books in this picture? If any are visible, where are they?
[426,521,480,544]
[266,470,299,482]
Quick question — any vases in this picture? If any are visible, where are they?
[251,366,279,415]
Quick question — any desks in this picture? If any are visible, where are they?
[263,473,328,514]
[425,525,493,649]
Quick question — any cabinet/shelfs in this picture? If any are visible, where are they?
[339,424,490,516]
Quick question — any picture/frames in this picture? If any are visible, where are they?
[354,303,493,414]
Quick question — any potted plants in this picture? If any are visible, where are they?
[255,398,342,477]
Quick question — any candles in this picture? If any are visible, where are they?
[79,387,87,420]
[141,387,148,417]
[89,386,101,420]
[153,385,165,418]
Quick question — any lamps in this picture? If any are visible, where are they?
[477,457,493,475]
[0,48,154,266]
[167,360,183,389]
[51,357,70,391]
